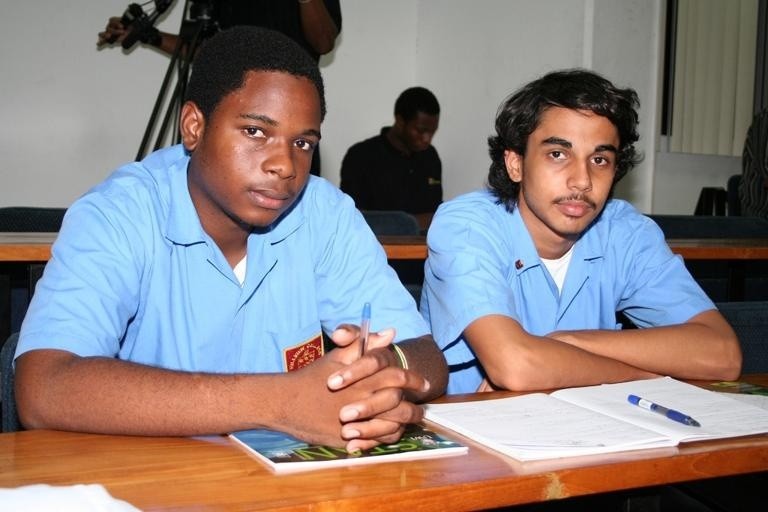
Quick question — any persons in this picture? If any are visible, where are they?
[97,2,344,177]
[340,87,442,284]
[11,23,449,455]
[418,69,744,393]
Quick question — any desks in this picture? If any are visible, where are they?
[1,371,768,510]
[0,232,54,302]
[377,236,429,262]
[665,238,768,302]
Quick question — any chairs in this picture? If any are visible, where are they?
[646,215,768,302]
[0,209,64,334]
[406,285,422,311]
[362,210,419,282]
[1,333,28,432]
[718,303,768,375]
[728,175,744,218]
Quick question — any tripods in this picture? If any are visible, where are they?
[120,0,223,162]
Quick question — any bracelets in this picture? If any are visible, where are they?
[389,342,409,370]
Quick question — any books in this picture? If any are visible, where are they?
[419,376,767,463]
[227,421,472,476]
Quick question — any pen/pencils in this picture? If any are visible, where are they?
[359,302,371,360]
[628,395,702,426]
